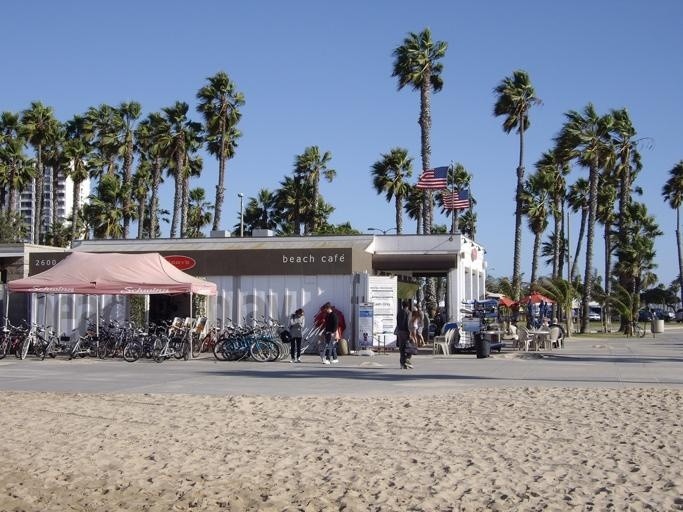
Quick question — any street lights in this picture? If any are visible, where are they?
[237,192,244,237]
[367,226,396,235]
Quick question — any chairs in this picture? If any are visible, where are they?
[501,325,564,351]
[433,327,456,355]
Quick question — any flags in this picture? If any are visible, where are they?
[443,191,471,209]
[414,166,448,190]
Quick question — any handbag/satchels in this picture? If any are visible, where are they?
[394,325,402,335]
[280,330,293,343]
[401,339,418,357]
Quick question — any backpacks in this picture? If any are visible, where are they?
[289,323,303,339]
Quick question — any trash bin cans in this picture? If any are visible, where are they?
[651,320,664,338]
[474,332,492,358]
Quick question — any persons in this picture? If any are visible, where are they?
[543,317,564,348]
[397,299,430,370]
[319,302,341,365]
[288,309,305,363]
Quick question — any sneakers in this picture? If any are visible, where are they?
[289,357,303,363]
[323,358,340,365]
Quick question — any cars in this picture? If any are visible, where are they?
[588,303,682,322]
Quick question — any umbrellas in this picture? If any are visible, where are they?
[495,291,556,307]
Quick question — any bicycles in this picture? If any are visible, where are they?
[0,315,291,365]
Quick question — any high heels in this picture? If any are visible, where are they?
[400,358,414,370]
